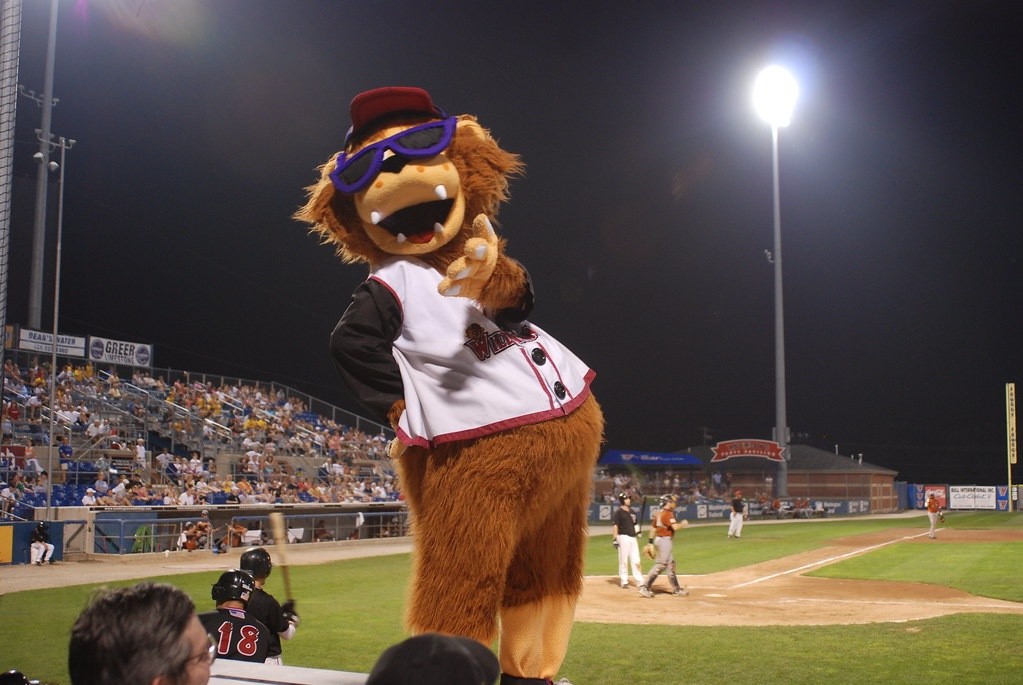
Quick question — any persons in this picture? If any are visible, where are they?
[724,472,732,488]
[364,633,499,685]
[772,497,786,519]
[759,492,766,507]
[66,582,216,685]
[796,497,813,518]
[593,470,710,503]
[193,569,282,665]
[728,490,745,539]
[0,356,404,551]
[925,494,944,539]
[712,470,721,490]
[639,494,689,597]
[30,522,54,565]
[611,493,645,588]
[239,547,301,664]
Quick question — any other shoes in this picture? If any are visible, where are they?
[639,588,652,598]
[673,589,688,595]
[623,584,628,588]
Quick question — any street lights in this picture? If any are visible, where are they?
[35,125,76,520]
[747,59,799,499]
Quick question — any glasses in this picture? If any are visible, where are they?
[187,634,217,665]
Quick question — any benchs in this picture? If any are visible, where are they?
[763,509,825,519]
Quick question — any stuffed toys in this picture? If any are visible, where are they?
[294,86,604,685]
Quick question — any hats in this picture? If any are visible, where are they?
[87,488,97,493]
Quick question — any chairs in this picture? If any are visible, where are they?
[13,485,164,517]
[297,411,348,435]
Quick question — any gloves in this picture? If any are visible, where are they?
[613,540,620,549]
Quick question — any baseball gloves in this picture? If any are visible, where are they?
[642,543,656,560]
[940,516,945,522]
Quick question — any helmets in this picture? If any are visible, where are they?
[619,493,632,505]
[662,495,675,506]
[212,569,255,610]
[241,548,272,579]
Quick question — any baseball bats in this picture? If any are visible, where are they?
[268,512,293,599]
[640,497,647,532]
